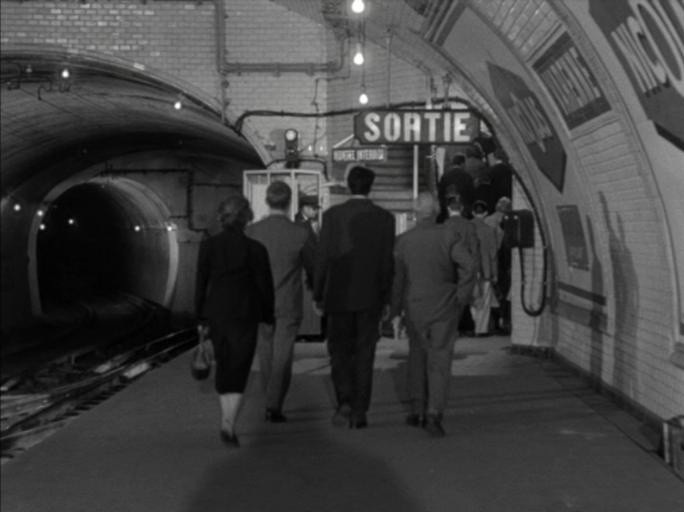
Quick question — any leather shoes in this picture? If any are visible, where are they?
[219,402,447,446]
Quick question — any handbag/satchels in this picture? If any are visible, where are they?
[190,335,211,382]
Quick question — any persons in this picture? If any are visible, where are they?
[193,197,276,447]
[389,193,476,434]
[436,130,517,340]
[242,179,315,424]
[313,164,396,431]
[293,194,322,228]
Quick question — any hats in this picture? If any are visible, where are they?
[299,194,322,209]
